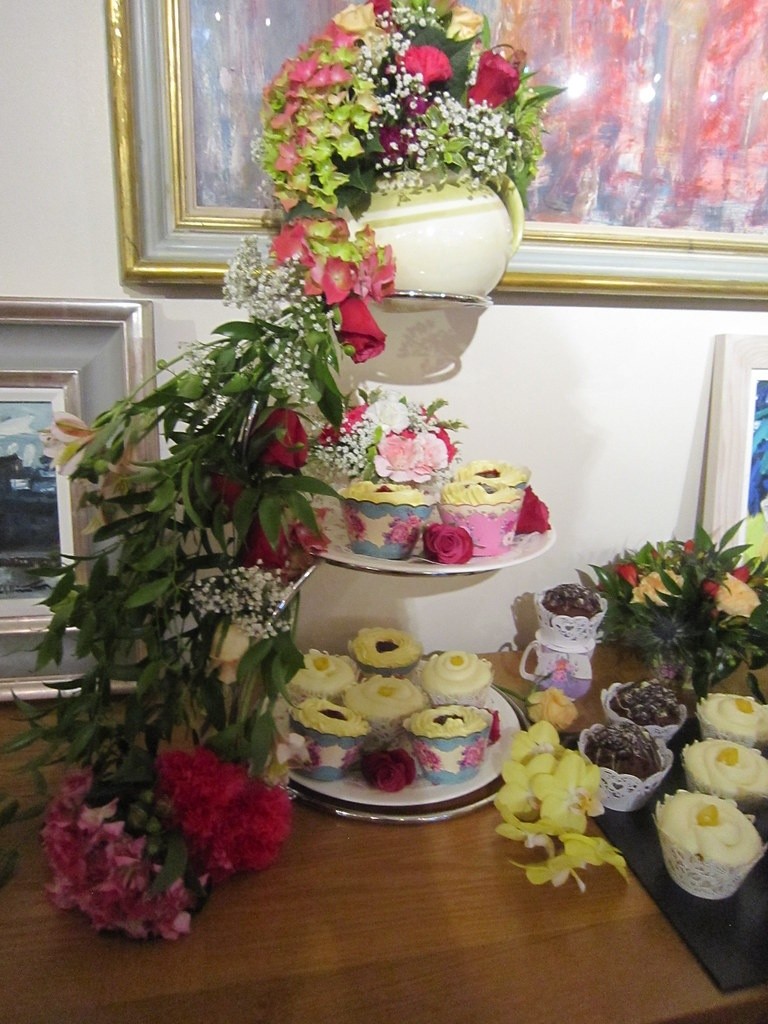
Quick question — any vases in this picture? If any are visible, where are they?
[651,649,714,692]
[336,166,510,304]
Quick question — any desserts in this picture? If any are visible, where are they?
[578,680,768,898]
[279,626,494,785]
[531,584,608,646]
[342,455,531,560]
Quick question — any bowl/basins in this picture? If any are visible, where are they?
[577,723,672,812]
[652,789,766,899]
[681,737,767,817]
[600,680,687,743]
[534,588,607,649]
[695,693,767,755]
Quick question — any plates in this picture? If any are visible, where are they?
[297,488,556,575]
[273,659,522,807]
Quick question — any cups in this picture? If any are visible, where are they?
[519,630,596,702]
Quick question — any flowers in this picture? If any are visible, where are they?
[254,0,566,295]
[492,665,628,898]
[581,518,768,705]
[0,243,472,948]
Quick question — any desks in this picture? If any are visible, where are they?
[0,647,768,1024]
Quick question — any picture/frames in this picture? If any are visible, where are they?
[103,1,768,308]
[0,294,174,704]
